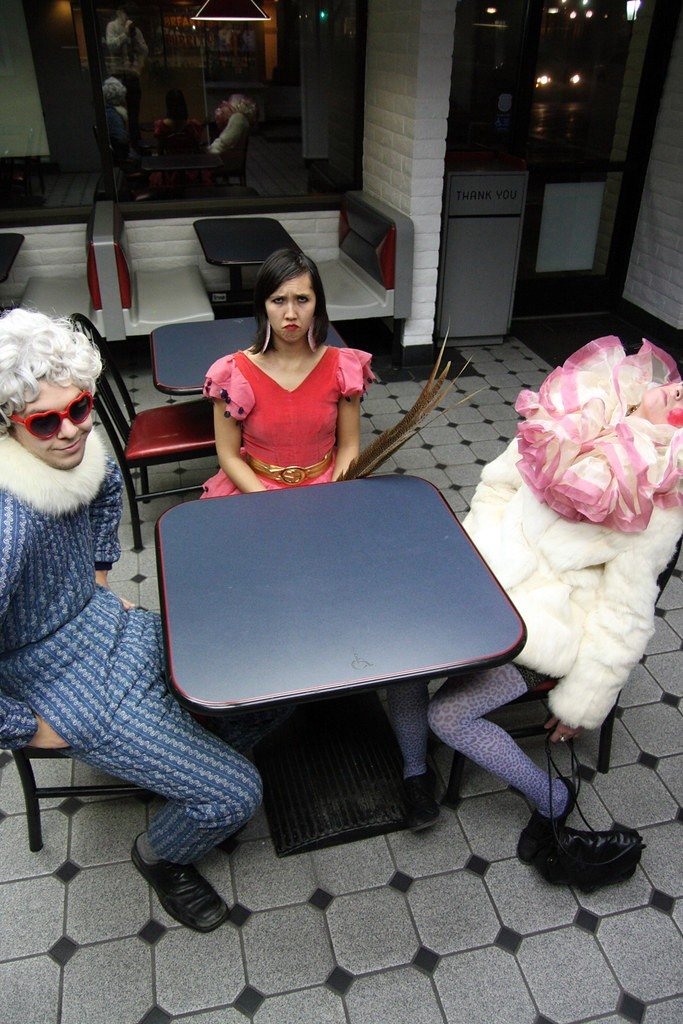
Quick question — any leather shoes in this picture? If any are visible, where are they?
[130,830,231,934]
[517,777,580,865]
[405,763,440,833]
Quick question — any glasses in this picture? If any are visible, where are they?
[9,389,94,439]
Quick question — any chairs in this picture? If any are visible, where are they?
[12,713,149,853]
[70,313,217,552]
[446,535,683,805]
[92,125,140,203]
[212,129,251,186]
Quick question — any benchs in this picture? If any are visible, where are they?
[105,201,214,374]
[313,189,415,366]
[18,201,111,380]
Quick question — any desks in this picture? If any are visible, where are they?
[137,186,259,199]
[153,474,528,859]
[142,154,225,198]
[0,233,24,285]
[194,218,304,316]
[150,315,349,398]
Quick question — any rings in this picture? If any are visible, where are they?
[560,736,565,742]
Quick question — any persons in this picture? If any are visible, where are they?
[105,4,149,122]
[208,93,258,155]
[149,91,218,188]
[0,308,262,931]
[195,251,376,500]
[376,334,683,864]
[103,77,140,175]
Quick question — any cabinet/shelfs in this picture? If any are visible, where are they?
[432,151,530,347]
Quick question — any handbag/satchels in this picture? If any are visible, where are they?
[533,727,647,888]
